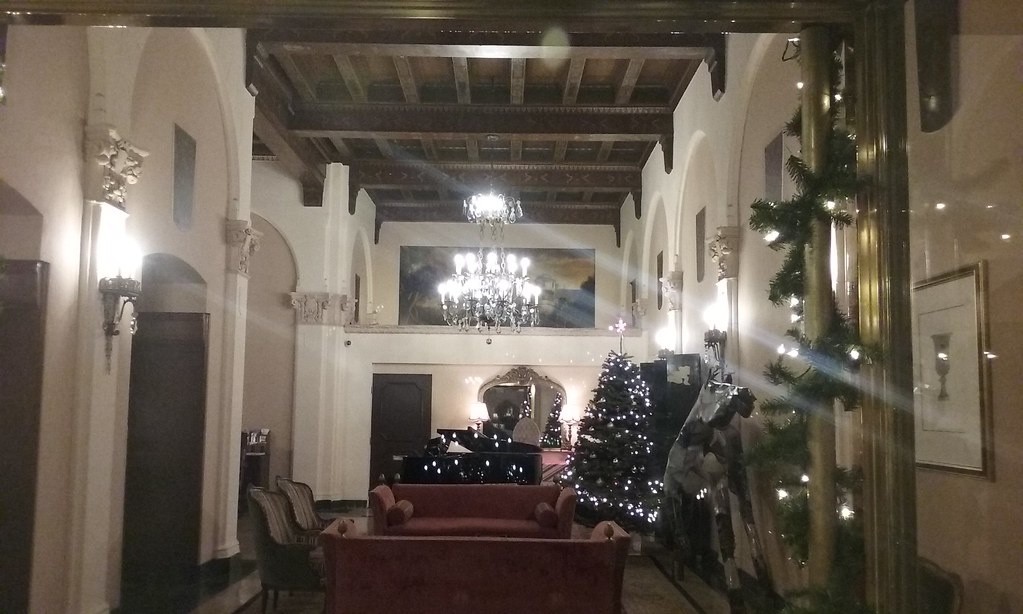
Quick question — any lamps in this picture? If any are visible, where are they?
[438,133,543,336]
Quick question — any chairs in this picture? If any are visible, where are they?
[246,482,330,614]
[275,476,336,596]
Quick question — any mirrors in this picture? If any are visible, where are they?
[478,368,566,442]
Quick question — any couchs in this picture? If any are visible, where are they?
[319,518,631,614]
[368,483,578,539]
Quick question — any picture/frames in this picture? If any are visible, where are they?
[908,258,997,484]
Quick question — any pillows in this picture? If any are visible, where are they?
[388,500,414,526]
[535,501,557,529]
